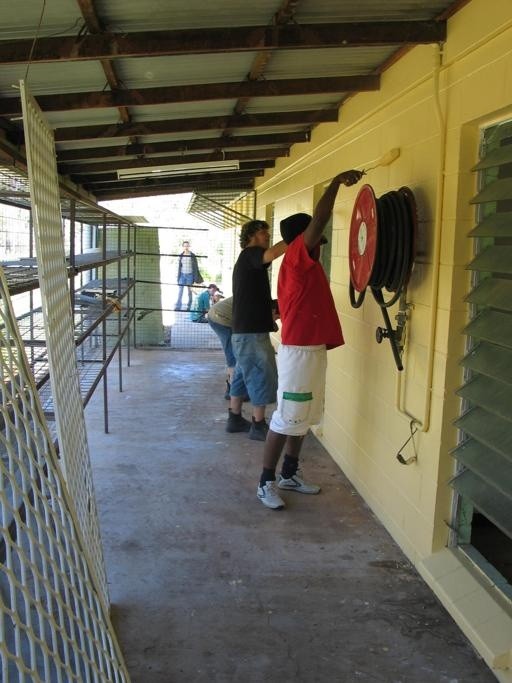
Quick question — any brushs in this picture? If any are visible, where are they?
[345,148,401,184]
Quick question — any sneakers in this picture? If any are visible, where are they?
[278,474,321,495]
[257,481,286,510]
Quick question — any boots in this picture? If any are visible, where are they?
[227,408,252,433]
[248,415,269,441]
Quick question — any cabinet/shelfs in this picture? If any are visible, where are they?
[1,143,136,564]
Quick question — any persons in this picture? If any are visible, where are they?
[207,296,280,401]
[209,288,225,306]
[224,220,287,442]
[173,241,203,311]
[256,169,362,509]
[190,284,218,323]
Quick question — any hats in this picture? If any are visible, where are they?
[214,291,225,298]
[280,213,312,245]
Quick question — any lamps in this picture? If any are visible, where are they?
[115,159,241,181]
